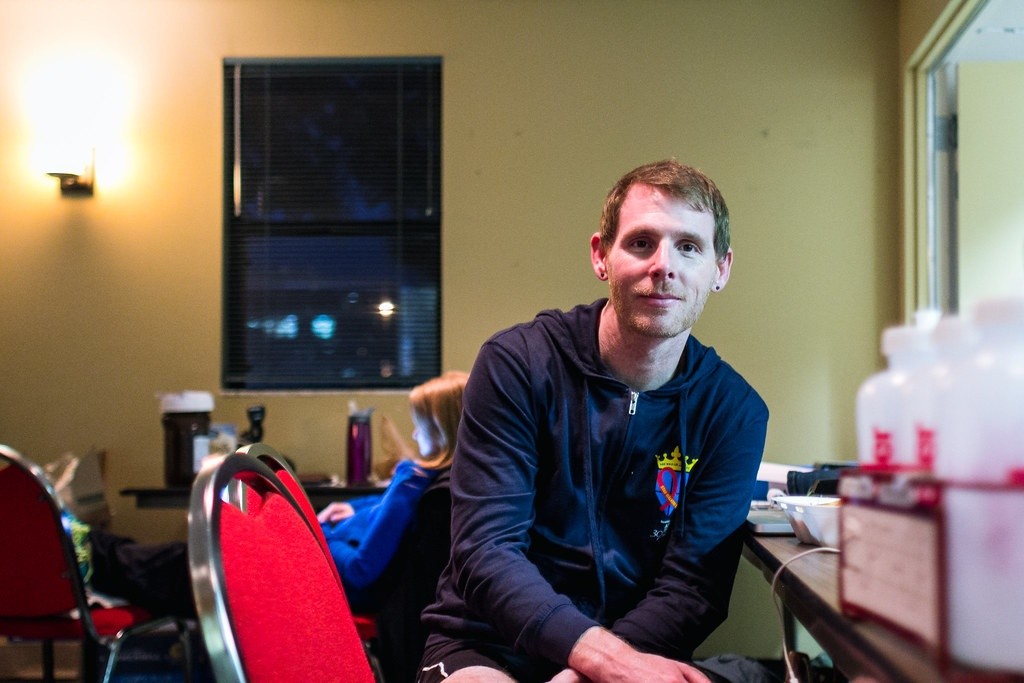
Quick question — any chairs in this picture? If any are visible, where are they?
[188,454,374,683]
[237,442,383,683]
[0,441,190,683]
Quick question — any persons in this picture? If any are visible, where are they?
[421,159,770,683]
[317,371,473,590]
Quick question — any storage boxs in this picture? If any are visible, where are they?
[833,475,1024,683]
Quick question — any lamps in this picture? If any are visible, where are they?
[47,134,95,196]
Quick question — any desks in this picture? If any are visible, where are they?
[740,534,1024,683]
[120,482,396,511]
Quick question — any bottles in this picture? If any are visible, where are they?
[347,403,374,486]
[854,297,1024,481]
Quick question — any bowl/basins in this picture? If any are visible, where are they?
[746,500,795,534]
[773,495,843,552]
